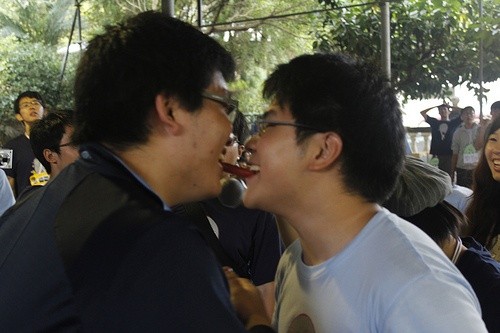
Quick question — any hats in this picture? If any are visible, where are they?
[381,157,448,219]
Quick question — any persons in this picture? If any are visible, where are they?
[451,106,482,190]
[242,53,487,333]
[0,169,17,217]
[0,91,50,199]
[475,101,500,150]
[169,111,281,321]
[421,104,464,177]
[382,155,453,217]
[17,109,80,201]
[0,9,275,333]
[412,203,500,333]
[465,114,500,261]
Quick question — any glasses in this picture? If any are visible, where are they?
[20,99,42,109]
[225,136,243,148]
[204,92,239,125]
[256,115,306,135]
